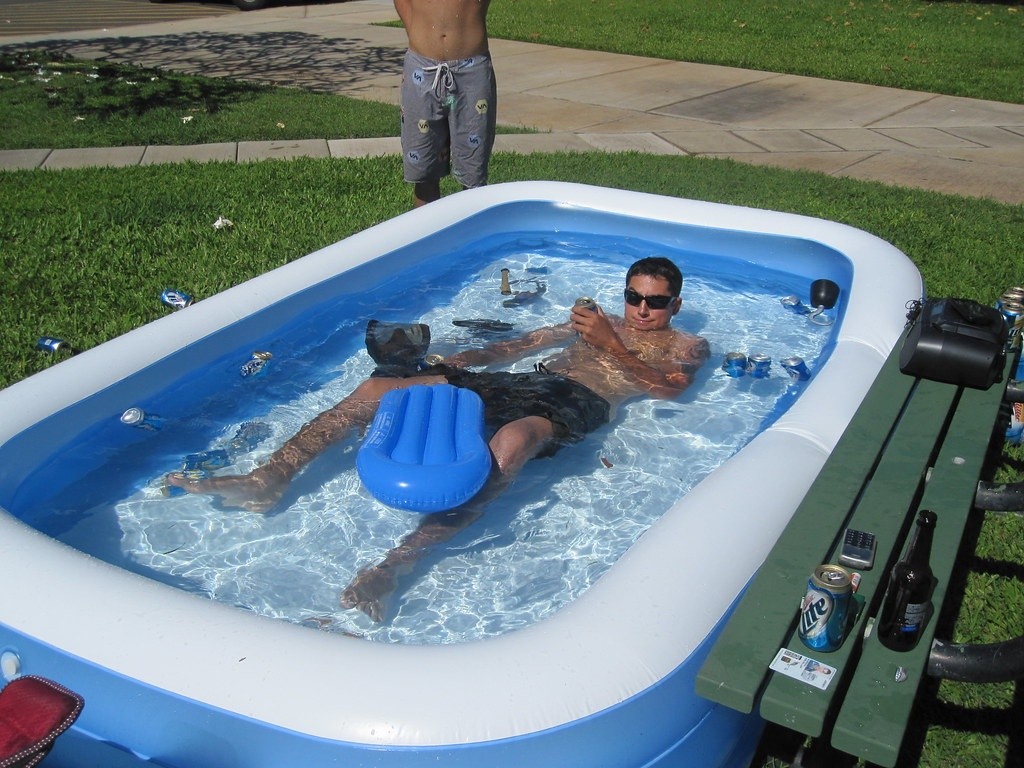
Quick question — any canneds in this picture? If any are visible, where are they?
[798,565,852,652]
[575,296,598,337]
[160,422,272,497]
[780,356,812,381]
[38,336,72,355]
[994,286,1024,353]
[161,288,195,309]
[747,353,771,379]
[415,355,443,373]
[120,407,163,431]
[240,350,272,377]
[726,352,746,378]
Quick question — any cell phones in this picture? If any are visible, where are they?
[950,299,993,324]
[839,528,876,571]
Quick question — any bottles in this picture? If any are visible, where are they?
[878,510,938,653]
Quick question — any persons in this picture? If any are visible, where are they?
[804,660,831,674]
[392,1,497,209]
[166,255,708,629]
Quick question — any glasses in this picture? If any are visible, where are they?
[624,289,677,309]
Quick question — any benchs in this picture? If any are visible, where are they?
[695,319,1024,768]
